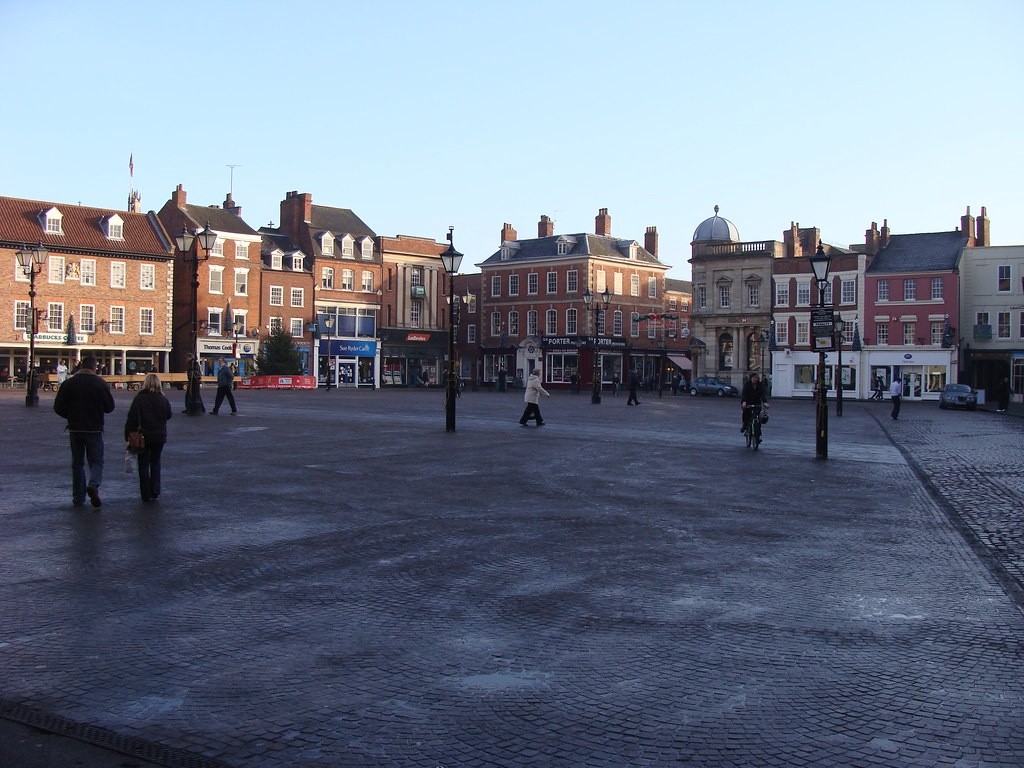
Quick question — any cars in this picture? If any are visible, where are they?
[690,376,739,397]
[939,384,978,411]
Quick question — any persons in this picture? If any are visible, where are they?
[54,356,116,507]
[210,356,237,415]
[644,372,663,398]
[125,374,173,502]
[182,353,205,414]
[671,374,686,395]
[627,366,642,406]
[499,367,508,393]
[996,378,1014,411]
[744,374,768,402]
[570,371,577,394]
[422,368,464,397]
[890,378,902,420]
[0,359,157,395]
[612,373,621,397]
[741,373,770,433]
[811,379,828,404]
[229,362,239,391]
[871,376,887,401]
[519,369,551,427]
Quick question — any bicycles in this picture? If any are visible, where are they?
[742,404,769,451]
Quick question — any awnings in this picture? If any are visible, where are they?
[667,356,692,370]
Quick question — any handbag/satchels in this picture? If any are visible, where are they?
[758,411,769,424]
[128,431,144,453]
[126,449,138,474]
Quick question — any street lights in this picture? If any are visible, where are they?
[325,313,335,391]
[439,241,464,433]
[758,331,767,382]
[175,220,217,414]
[834,311,845,417]
[233,322,242,391]
[808,238,832,460]
[581,286,614,405]
[15,237,50,408]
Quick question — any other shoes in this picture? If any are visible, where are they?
[209,411,218,415]
[740,427,745,433]
[182,409,187,413]
[72,498,83,505]
[142,492,158,501]
[520,422,528,426]
[87,486,101,508]
[202,409,205,413]
[230,411,237,415]
[537,422,545,426]
